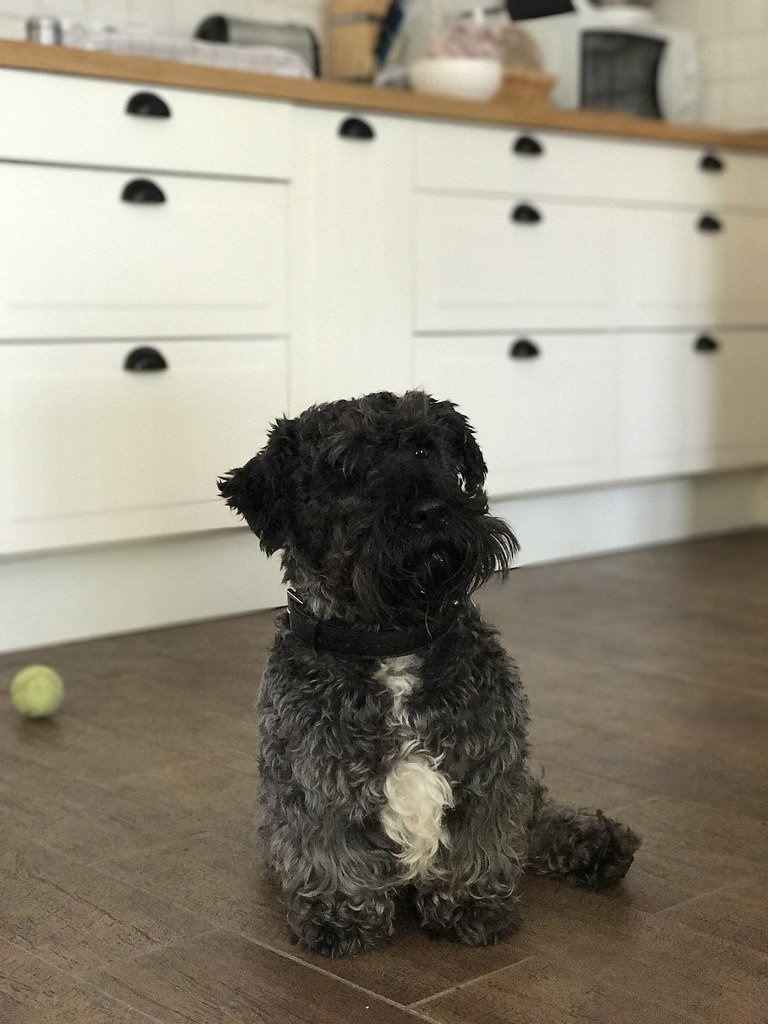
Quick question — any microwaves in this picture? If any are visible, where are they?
[498,4,699,125]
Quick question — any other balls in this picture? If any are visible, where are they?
[9,664,64,719]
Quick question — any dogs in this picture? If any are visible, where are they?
[218,392,643,955]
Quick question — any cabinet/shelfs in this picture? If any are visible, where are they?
[0,38,768,655]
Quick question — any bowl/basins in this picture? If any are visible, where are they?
[408,57,502,101]
